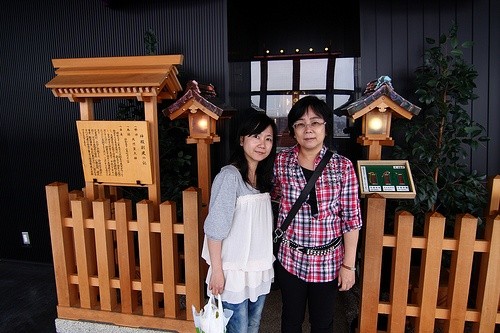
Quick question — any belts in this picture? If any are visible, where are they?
[280,236,342,256]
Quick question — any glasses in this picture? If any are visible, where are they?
[292,121,327,130]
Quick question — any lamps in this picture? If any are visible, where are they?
[168,82,222,143]
[333,76,421,145]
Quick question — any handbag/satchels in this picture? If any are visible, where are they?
[272,233,284,290]
[192,292,234,333]
